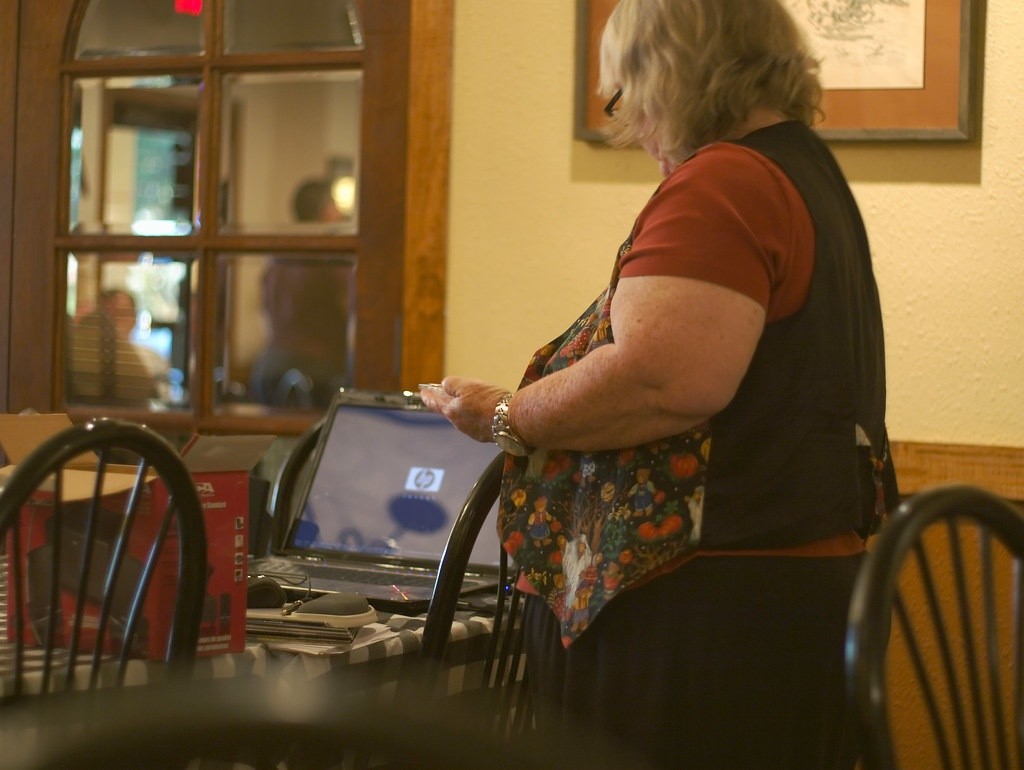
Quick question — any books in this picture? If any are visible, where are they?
[246,607,362,647]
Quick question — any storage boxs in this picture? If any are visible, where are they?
[0,413,277,663]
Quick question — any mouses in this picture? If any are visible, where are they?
[245,574,287,609]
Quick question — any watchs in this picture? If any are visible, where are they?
[491,393,537,457]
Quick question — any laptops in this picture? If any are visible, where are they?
[241,390,516,611]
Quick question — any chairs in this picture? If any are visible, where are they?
[0,419,207,714]
[417,450,578,743]
[843,483,1024,770]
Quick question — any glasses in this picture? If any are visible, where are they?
[604,90,625,120]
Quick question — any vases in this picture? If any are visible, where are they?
[572,0,985,145]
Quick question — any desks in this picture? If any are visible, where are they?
[1,595,529,708]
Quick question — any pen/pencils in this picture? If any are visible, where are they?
[282,597,312,616]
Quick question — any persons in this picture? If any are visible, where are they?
[417,0,902,770]
[246,183,353,406]
[100,288,170,397]
